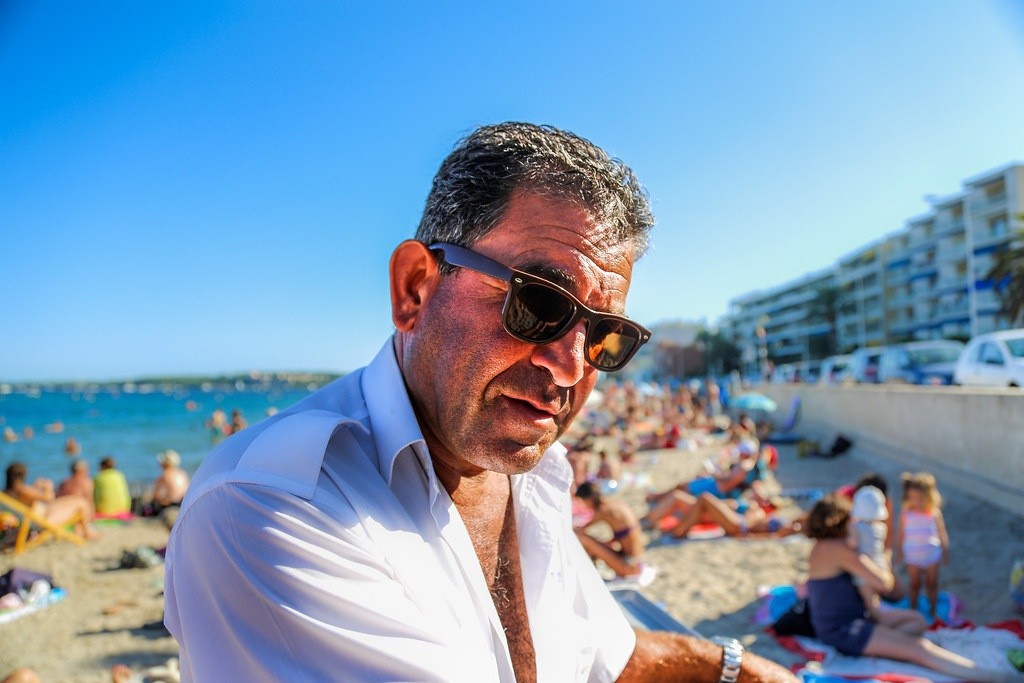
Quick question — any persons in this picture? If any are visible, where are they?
[0,400,247,616]
[162,122,802,683]
[569,377,1022,683]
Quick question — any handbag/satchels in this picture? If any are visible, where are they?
[0,568,52,597]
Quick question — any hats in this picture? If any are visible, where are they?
[737,441,756,455]
[156,449,180,467]
[850,486,889,520]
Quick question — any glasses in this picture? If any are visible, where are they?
[428,242,651,372]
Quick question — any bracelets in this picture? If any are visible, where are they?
[711,634,746,683]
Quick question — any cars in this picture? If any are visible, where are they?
[952,328,1024,388]
[877,338,968,387]
[769,327,888,385]
